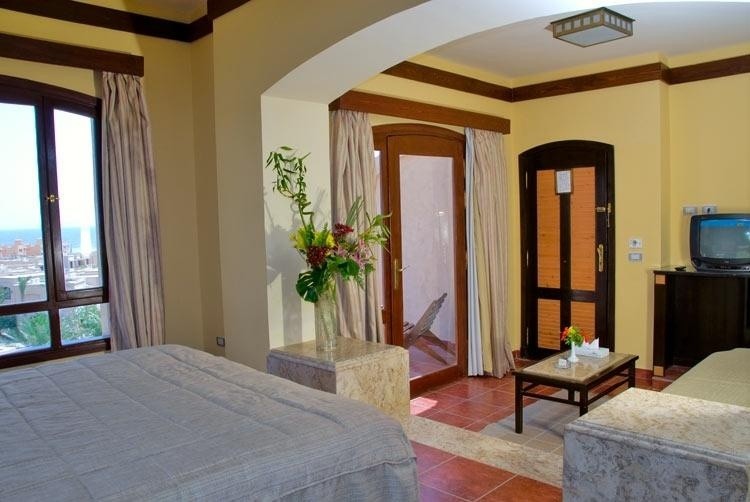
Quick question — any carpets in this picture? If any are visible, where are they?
[478,388,613,456]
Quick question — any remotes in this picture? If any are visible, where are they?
[675,266,686,271]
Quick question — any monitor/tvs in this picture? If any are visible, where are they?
[689,213,750,273]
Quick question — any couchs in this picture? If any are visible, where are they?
[563,348,750,502]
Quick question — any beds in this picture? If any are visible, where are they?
[0,342,420,502]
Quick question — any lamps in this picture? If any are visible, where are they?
[550,7,636,48]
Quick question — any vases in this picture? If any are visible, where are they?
[568,342,578,362]
[314,293,337,350]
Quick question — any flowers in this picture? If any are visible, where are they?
[562,323,583,348]
[266,146,393,303]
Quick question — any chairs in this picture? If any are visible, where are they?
[403,293,456,366]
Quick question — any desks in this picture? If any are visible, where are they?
[653,271,750,377]
[266,336,411,438]
